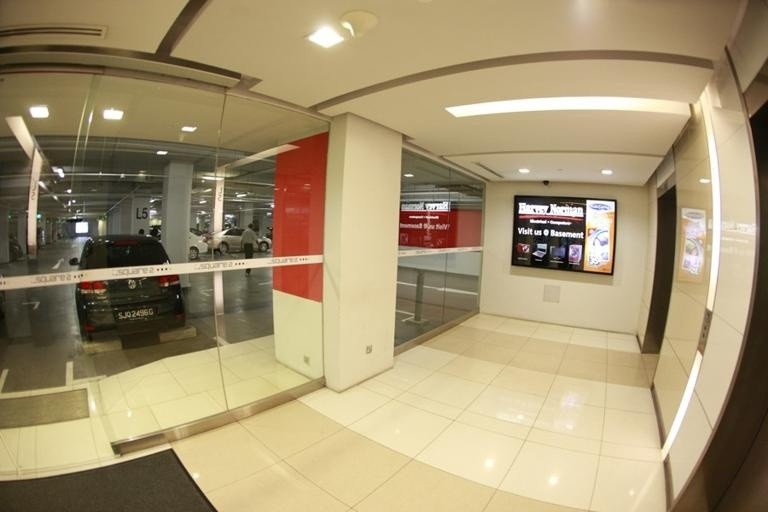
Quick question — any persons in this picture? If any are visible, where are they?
[240,223,259,276]
[154,226,161,237]
[139,229,144,234]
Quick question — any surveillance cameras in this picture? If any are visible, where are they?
[543,180,549,186]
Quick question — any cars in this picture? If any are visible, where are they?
[69,234,186,344]
[149,219,272,261]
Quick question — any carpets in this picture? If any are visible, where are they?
[0,448,217,512]
